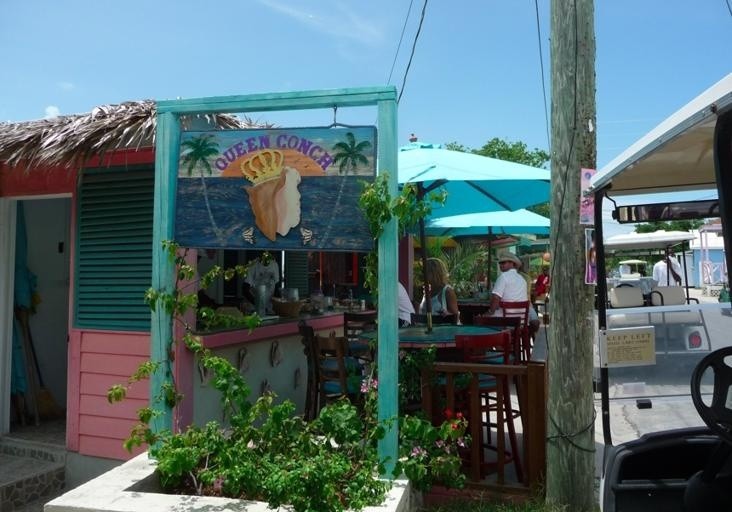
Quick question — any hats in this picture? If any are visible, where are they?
[498,250,522,267]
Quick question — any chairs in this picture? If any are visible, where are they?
[652,286,686,305]
[608,288,645,308]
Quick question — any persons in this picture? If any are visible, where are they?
[397,283,416,329]
[418,258,463,327]
[475,248,548,353]
[242,250,282,316]
[652,248,683,287]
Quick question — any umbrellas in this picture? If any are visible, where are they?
[376,131,553,327]
[404,207,550,291]
